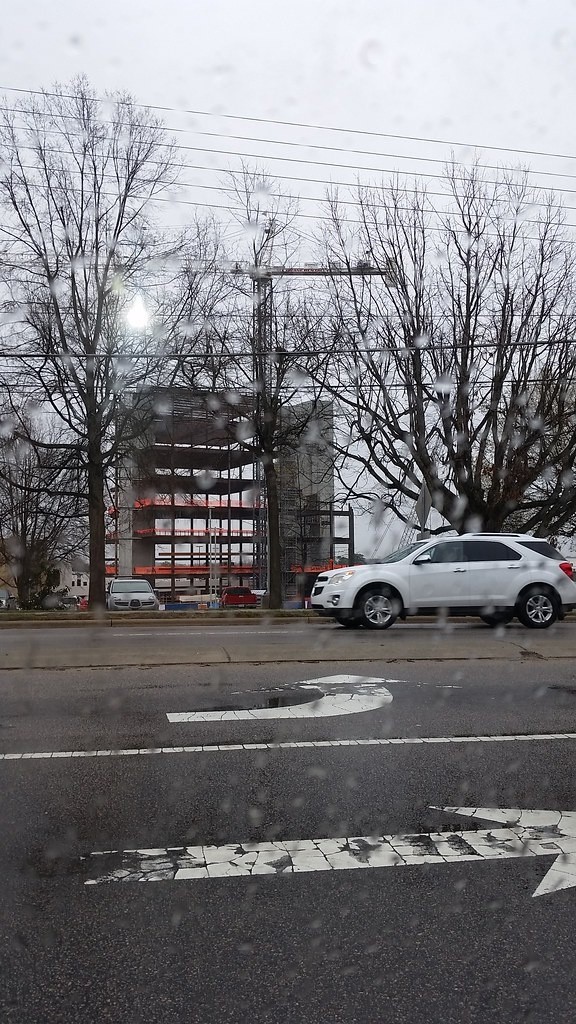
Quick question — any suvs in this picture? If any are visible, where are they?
[309,532,576,630]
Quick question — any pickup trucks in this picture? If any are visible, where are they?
[222,586,257,609]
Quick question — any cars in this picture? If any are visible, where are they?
[106,578,160,611]
[62,595,89,612]
